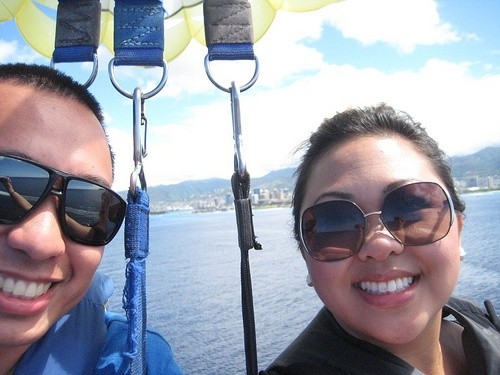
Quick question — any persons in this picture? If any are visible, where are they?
[0,64,182,375]
[258,106,499,374]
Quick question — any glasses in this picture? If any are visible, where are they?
[300,182,453,262]
[0,155,126,246]
